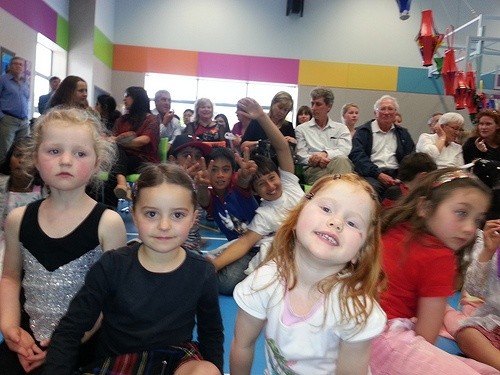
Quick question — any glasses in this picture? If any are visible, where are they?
[447,124,464,134]
[123,93,130,98]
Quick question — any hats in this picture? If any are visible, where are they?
[167,135,213,158]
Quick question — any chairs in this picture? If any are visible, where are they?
[96,170,110,202]
[121,174,141,213]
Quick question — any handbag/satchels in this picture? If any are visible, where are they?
[474,160,500,186]
[257,140,294,162]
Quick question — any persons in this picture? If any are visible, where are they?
[368,166,500,375]
[0,57,30,161]
[46,163,225,374]
[209,99,306,297]
[456,219,500,370]
[231,172,387,375]
[0,75,500,241]
[0,107,127,374]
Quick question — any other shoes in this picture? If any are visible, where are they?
[114,186,132,202]
[207,213,213,221]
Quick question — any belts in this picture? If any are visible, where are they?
[3,111,25,120]
